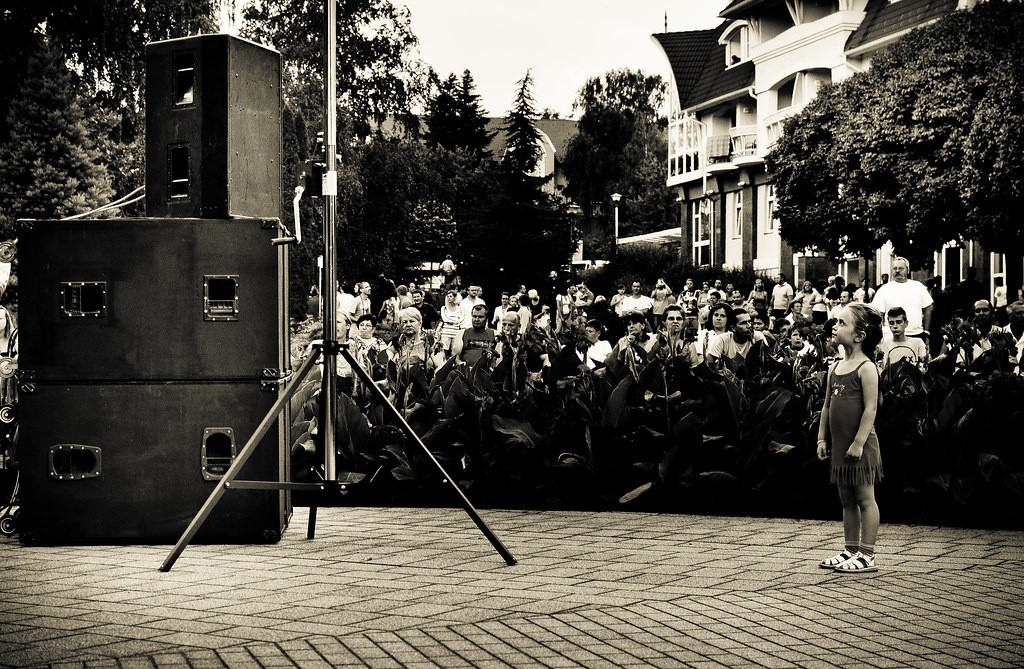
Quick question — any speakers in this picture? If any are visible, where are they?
[145,34,284,220]
[16,377,293,545]
[15,219,292,379]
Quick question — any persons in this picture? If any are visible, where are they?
[817,302,885,573]
[0,305,21,472]
[290,254,1024,481]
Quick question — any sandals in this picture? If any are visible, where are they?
[818,548,878,572]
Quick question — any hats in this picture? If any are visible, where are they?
[894,257,910,274]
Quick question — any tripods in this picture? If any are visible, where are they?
[158,0,519,572]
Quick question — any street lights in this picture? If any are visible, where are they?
[611,193,622,247]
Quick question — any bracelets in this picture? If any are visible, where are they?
[817,440,826,445]
